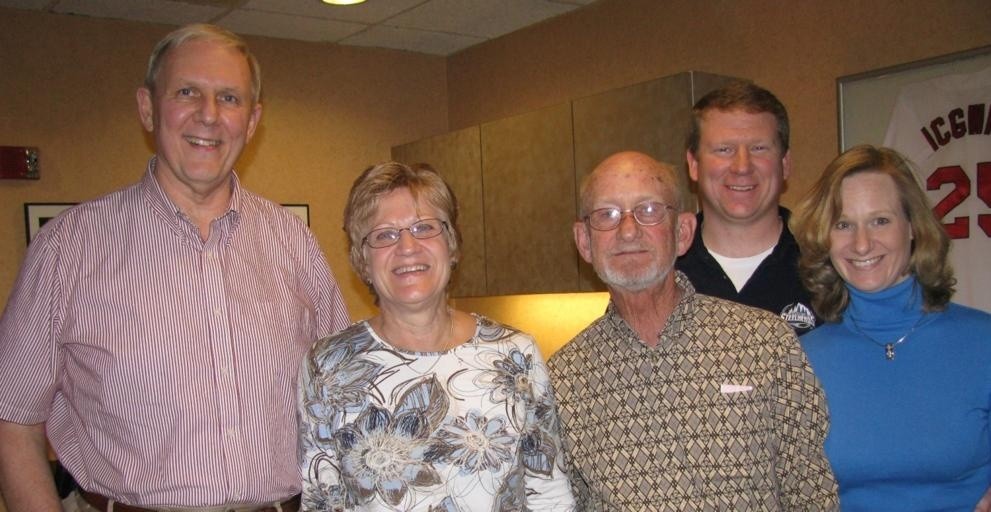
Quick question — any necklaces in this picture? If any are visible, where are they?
[848,302,927,361]
[379,309,453,374]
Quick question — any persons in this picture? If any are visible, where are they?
[788,145,991,512]
[545,152,840,512]
[297,161,577,512]
[674,80,826,338]
[0,24,354,512]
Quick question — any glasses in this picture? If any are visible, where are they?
[360,218,453,249]
[583,200,679,232]
